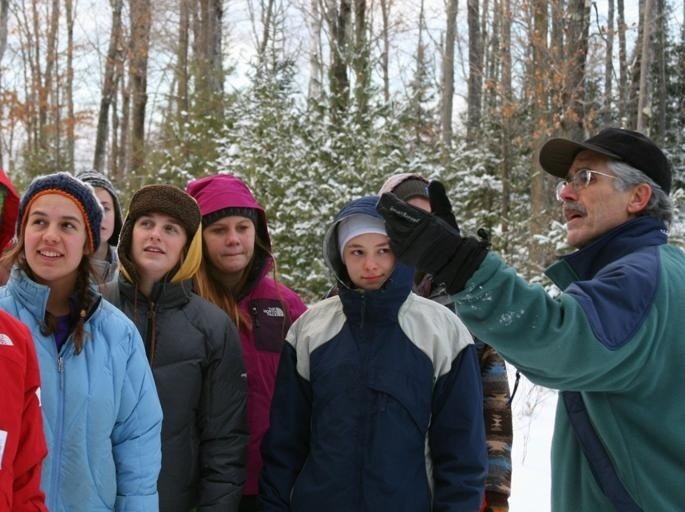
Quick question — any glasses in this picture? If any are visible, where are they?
[555,168,615,202]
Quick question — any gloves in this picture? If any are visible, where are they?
[377,181,488,294]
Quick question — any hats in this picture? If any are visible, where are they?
[116,185,203,285]
[539,127,673,195]
[202,208,258,230]
[77,168,122,247]
[393,177,431,201]
[17,172,102,254]
[337,215,388,262]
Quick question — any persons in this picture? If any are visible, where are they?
[92,184,248,511]
[0,166,51,512]
[0,173,164,511]
[376,127,685,511]
[73,170,124,285]
[185,173,310,511]
[376,174,513,512]
[258,196,488,512]
